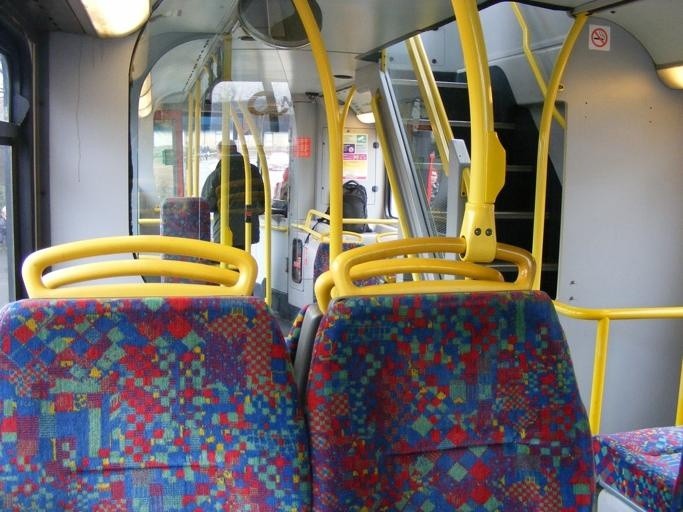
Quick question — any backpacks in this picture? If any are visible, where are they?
[304,180,372,243]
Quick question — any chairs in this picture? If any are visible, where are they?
[0,197,683,512]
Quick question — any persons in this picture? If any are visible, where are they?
[202,141,266,251]
[274,167,288,200]
